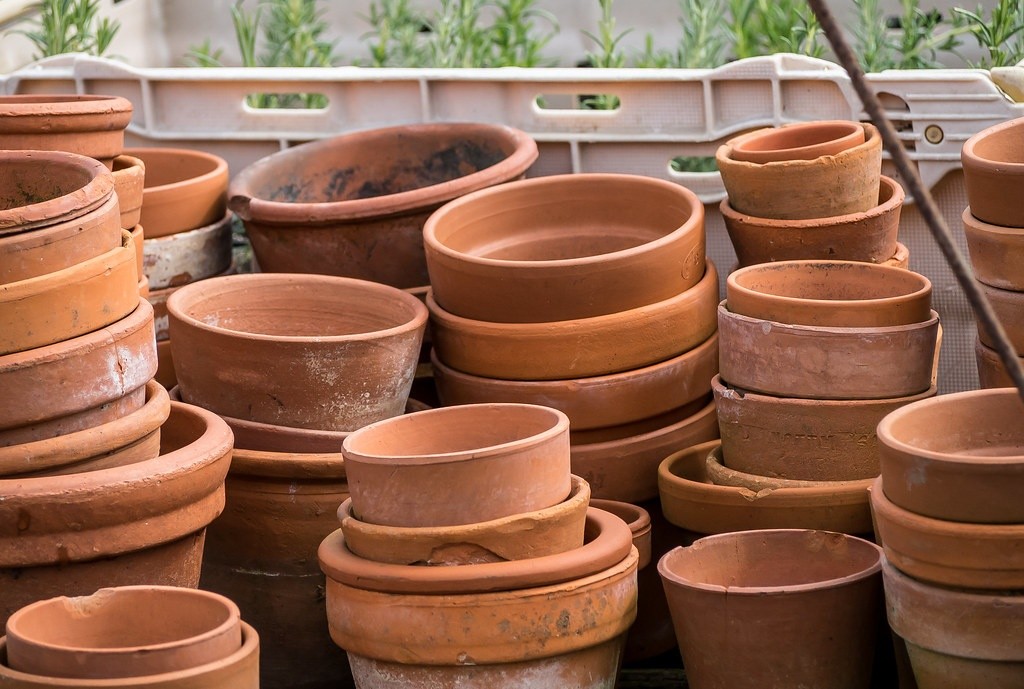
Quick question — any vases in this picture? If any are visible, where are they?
[0,94,1024,689]
